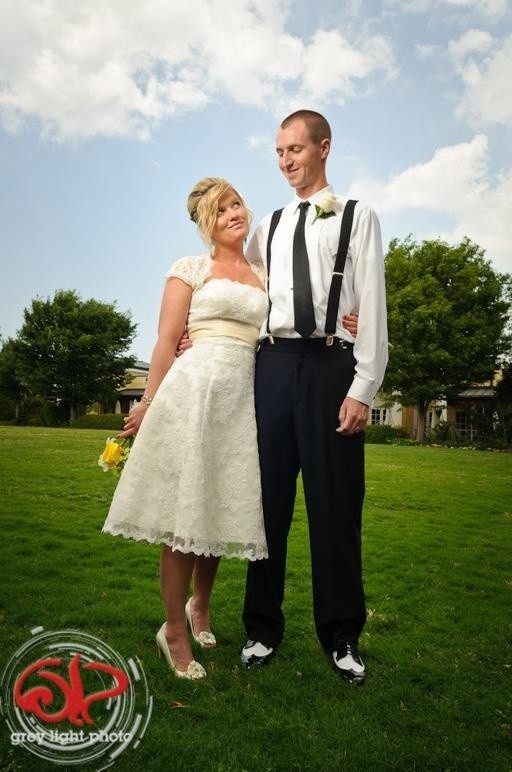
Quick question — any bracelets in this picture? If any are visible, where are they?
[140,397,153,406]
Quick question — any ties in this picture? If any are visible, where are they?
[292,201,317,338]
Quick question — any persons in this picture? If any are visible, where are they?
[97,174,369,682]
[171,110,396,686]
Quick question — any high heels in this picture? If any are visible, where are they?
[156,622,206,679]
[179,595,216,649]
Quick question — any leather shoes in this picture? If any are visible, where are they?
[241,637,274,668]
[330,640,367,685]
